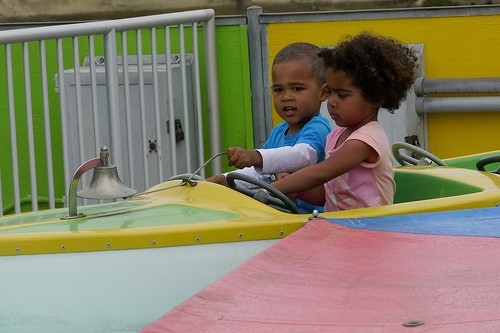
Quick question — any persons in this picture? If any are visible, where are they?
[264,29,419,214]
[200,41,335,216]
